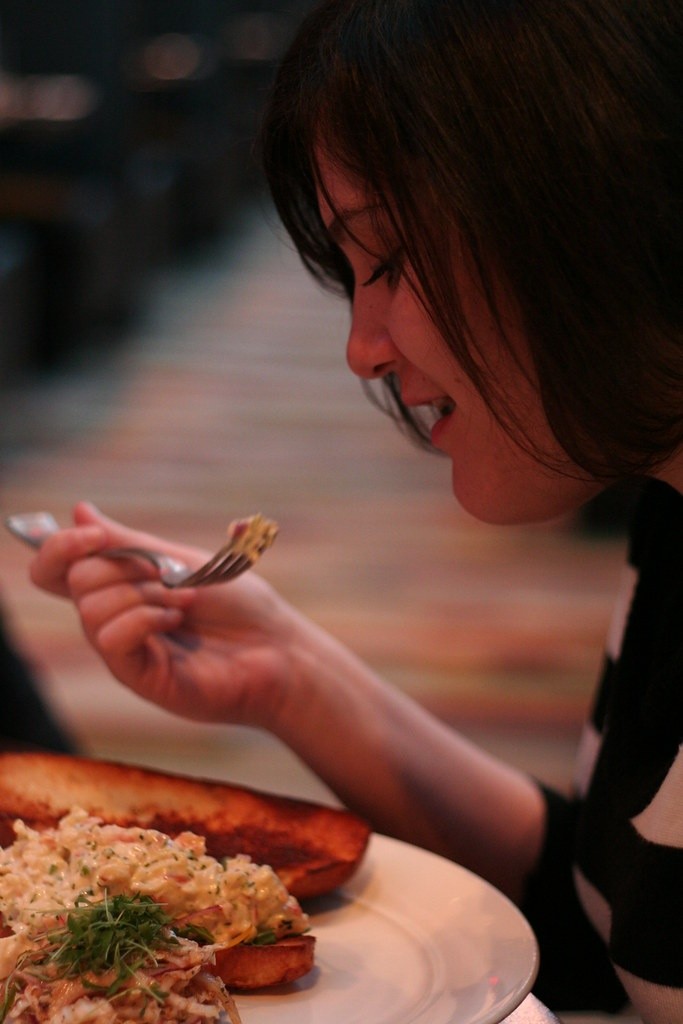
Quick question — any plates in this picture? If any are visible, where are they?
[235,824,540,1024]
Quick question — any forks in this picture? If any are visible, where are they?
[7,511,278,590]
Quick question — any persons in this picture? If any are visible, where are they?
[30,0,681,1024]
